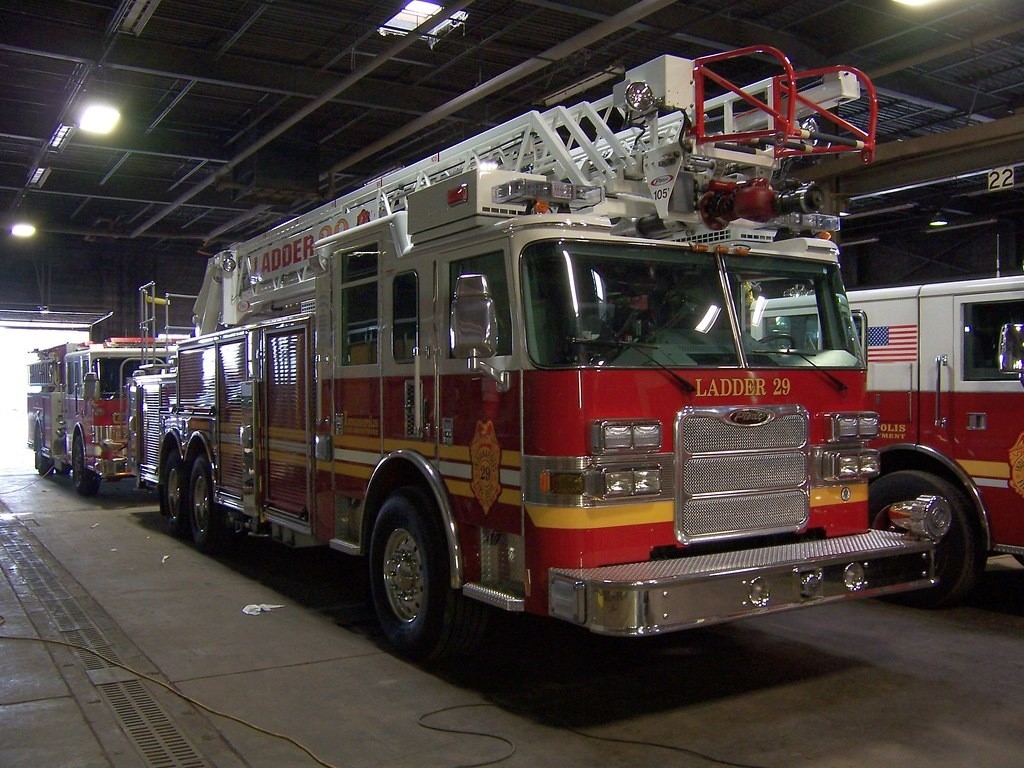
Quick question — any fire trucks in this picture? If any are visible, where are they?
[27,333,192,495]
[742,277,1024,614]
[118,44,954,669]
